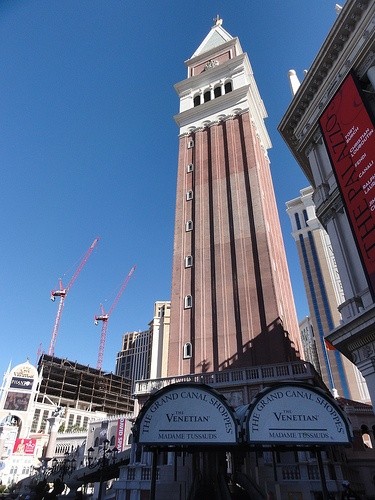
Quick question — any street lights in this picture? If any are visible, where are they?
[87,437,121,500]
[51,451,77,500]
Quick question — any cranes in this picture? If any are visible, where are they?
[49,238,101,355]
[92,264,139,370]
